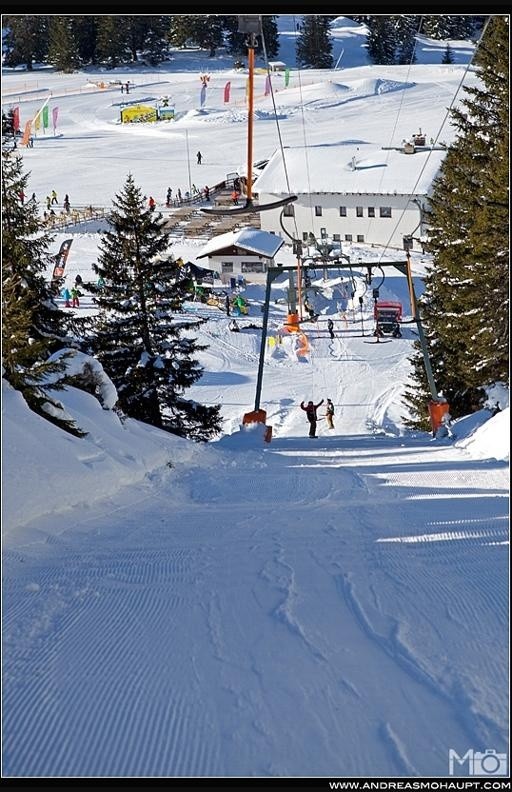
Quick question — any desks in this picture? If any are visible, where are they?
[163,191,261,236]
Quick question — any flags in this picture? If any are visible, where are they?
[199,68,289,106]
[12,106,60,148]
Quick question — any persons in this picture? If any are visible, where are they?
[223,295,231,316]
[166,176,260,207]
[17,187,71,221]
[232,294,249,316]
[197,150,203,165]
[327,318,335,339]
[300,399,324,438]
[323,398,336,429]
[228,319,240,332]
[149,196,155,213]
[336,300,343,313]
[308,308,319,324]
[55,263,106,310]
